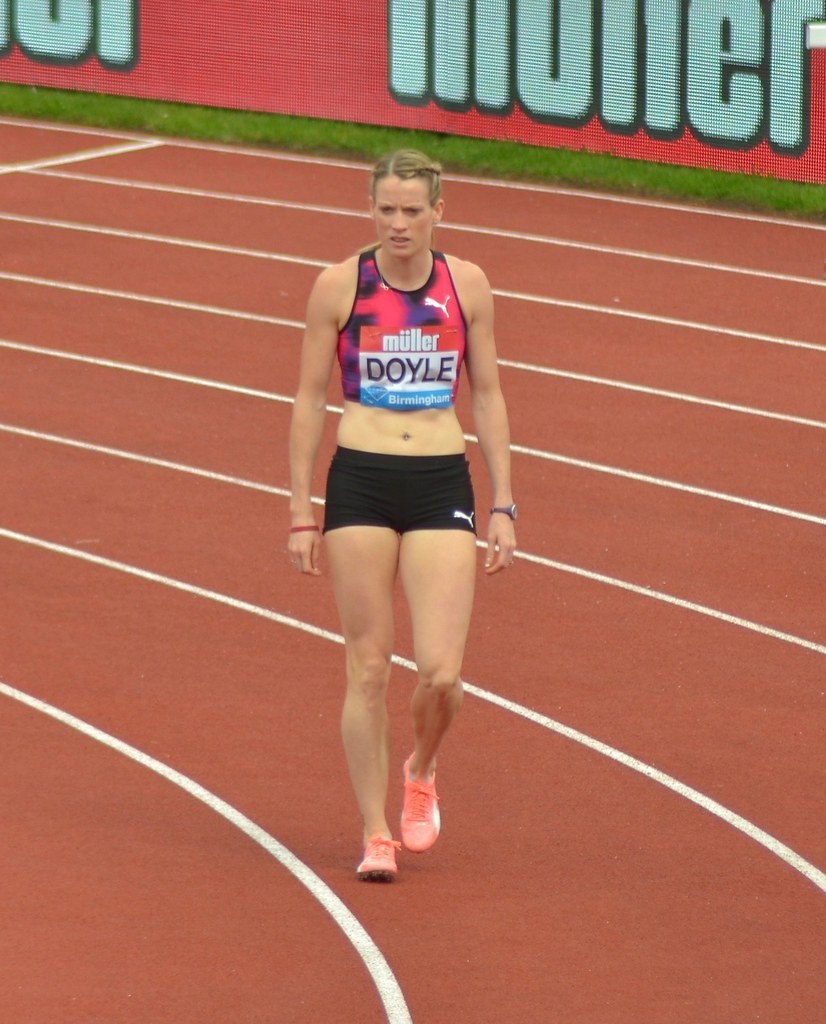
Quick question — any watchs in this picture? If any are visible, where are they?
[490,504,520,520]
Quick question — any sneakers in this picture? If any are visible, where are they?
[400,749,441,854]
[357,836,402,881]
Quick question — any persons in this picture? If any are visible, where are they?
[288,150,518,882]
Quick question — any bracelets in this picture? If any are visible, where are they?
[289,526,320,533]
